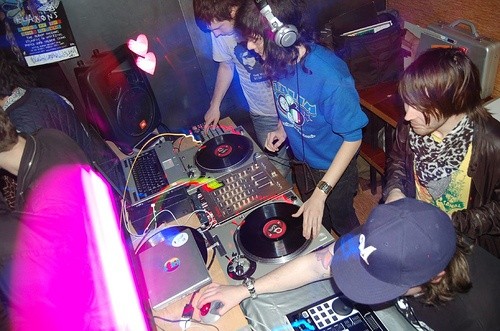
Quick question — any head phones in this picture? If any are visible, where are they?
[254,0,298,48]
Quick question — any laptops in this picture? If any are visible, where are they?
[89,125,190,206]
[139,228,213,311]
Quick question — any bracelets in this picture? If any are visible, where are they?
[316,180,333,196]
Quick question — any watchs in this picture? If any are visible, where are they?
[242,276,258,299]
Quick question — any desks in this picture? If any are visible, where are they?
[105,116,249,331]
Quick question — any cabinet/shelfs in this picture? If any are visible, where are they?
[359,97,406,197]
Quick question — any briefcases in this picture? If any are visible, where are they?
[415,18,500,100]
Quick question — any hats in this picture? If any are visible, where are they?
[331,197,457,305]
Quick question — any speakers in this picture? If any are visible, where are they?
[73,43,162,148]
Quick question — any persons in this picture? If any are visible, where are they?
[380,47,500,256]
[192,0,371,242]
[0,59,109,322]
[191,197,500,331]
